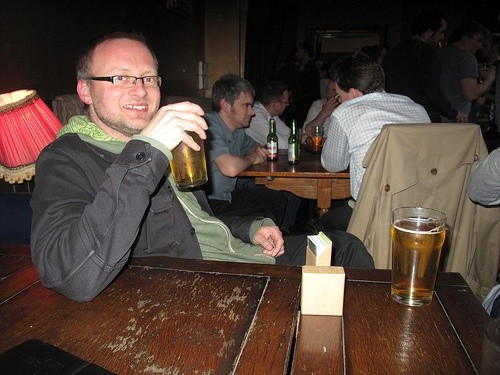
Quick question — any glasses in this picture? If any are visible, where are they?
[335,93,349,99]
[281,101,290,106]
[83,76,161,88]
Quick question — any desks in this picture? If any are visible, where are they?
[236,149,351,216]
[0,242,500,375]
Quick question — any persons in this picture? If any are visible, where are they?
[244,78,292,150]
[201,73,301,236]
[437,21,496,115]
[382,15,469,123]
[480,34,500,113]
[30,33,375,301]
[299,64,342,139]
[295,43,321,129]
[315,50,432,232]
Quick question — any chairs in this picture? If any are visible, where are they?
[345,123,500,303]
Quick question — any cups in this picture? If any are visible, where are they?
[167,126,209,192]
[391,207,447,307]
[312,126,323,152]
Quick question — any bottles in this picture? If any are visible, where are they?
[267,119,278,160]
[288,119,300,164]
